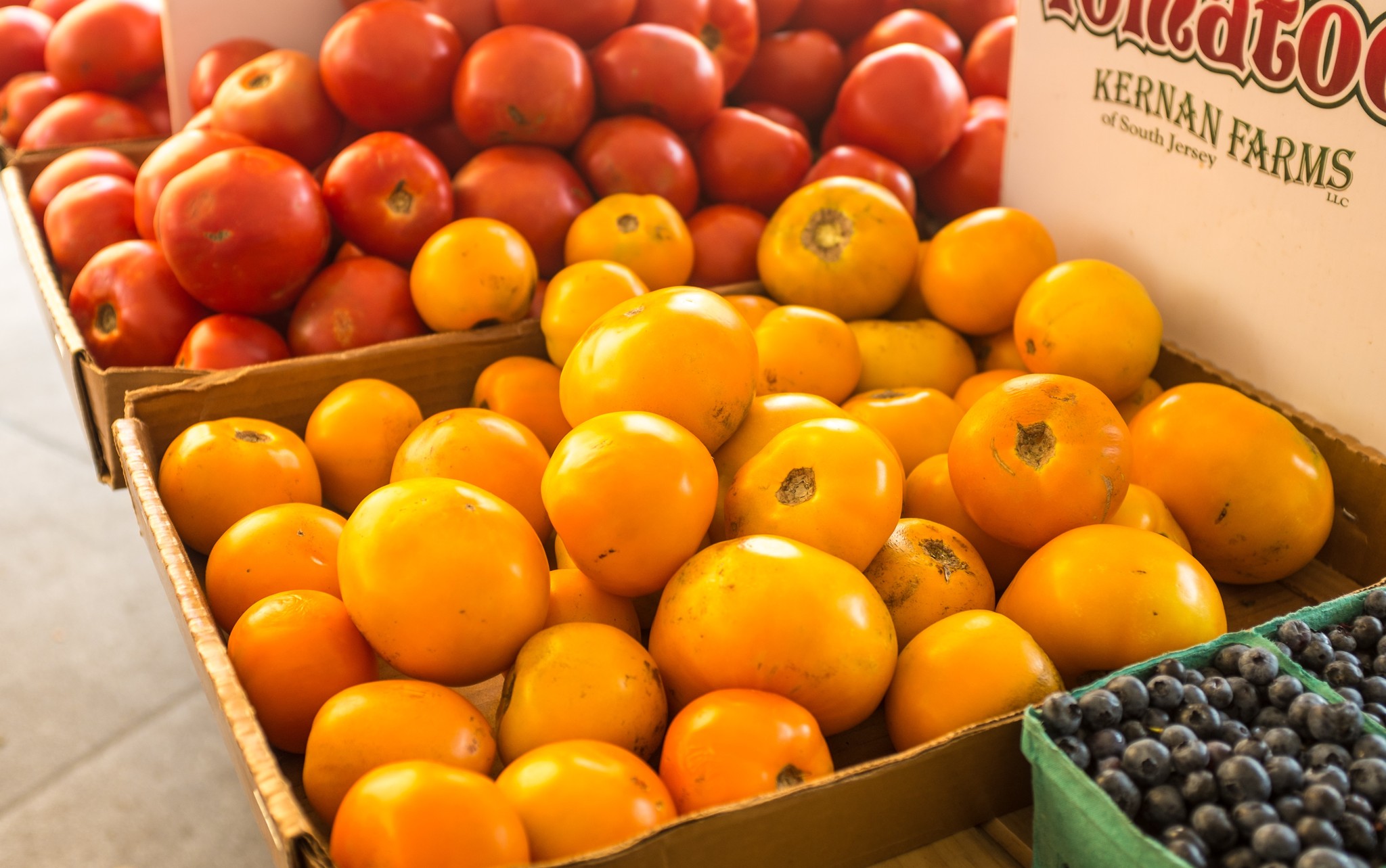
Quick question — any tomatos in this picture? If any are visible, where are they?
[0,0,1020,371]
[157,176,1332,868]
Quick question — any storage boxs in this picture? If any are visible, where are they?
[0,134,1386,868]
